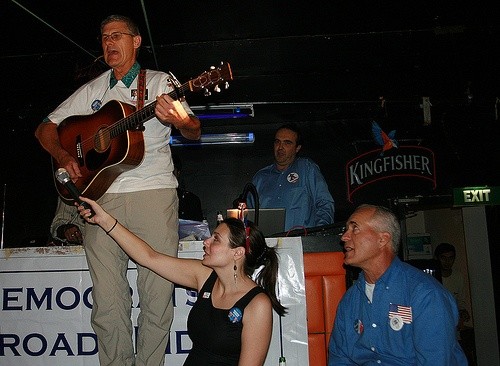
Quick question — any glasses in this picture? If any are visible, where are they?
[97,32,135,43]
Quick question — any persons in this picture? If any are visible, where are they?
[74,196,289,366]
[131,90,138,100]
[327,204,468,366]
[35,16,202,366]
[433,242,476,366]
[246,126,335,231]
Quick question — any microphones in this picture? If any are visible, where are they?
[237,182,259,227]
[54,168,97,219]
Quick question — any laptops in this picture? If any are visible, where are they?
[226,208,287,237]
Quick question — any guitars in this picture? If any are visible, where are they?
[51,60,234,206]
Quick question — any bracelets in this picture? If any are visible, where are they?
[107,219,118,233]
